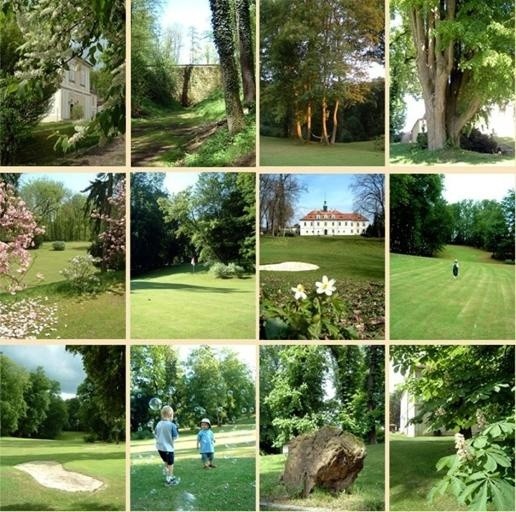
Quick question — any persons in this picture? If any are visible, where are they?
[451,258,460,279]
[153,405,183,488]
[190,256,196,272]
[195,417,219,471]
[158,260,163,272]
[496,145,502,158]
[163,259,168,272]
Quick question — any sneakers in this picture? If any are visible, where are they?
[164,475,180,488]
[202,464,217,470]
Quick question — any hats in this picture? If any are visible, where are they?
[199,418,211,428]
